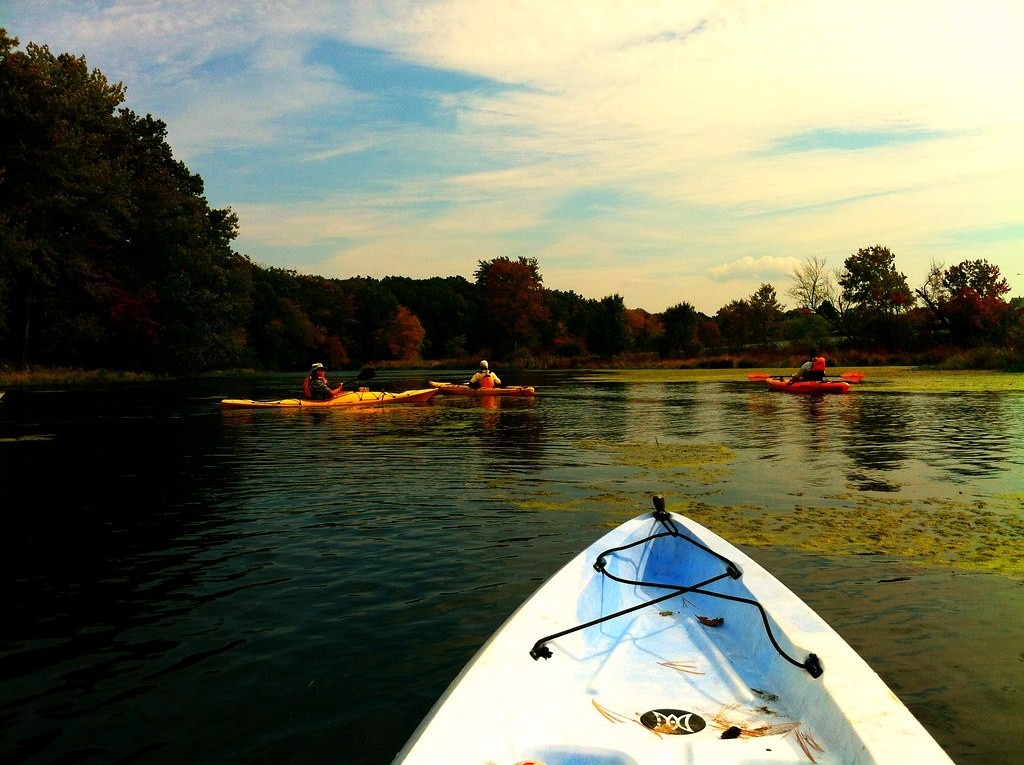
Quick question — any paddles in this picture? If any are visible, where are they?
[747,371,865,383]
[332,367,377,389]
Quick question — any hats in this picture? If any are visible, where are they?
[308,363,328,373]
[809,347,819,356]
[480,360,488,368]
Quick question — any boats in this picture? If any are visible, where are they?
[765,378,850,394]
[220,388,438,411]
[428,380,535,397]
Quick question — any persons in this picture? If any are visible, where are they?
[791,347,825,381]
[304,363,344,401]
[466,359,502,388]
